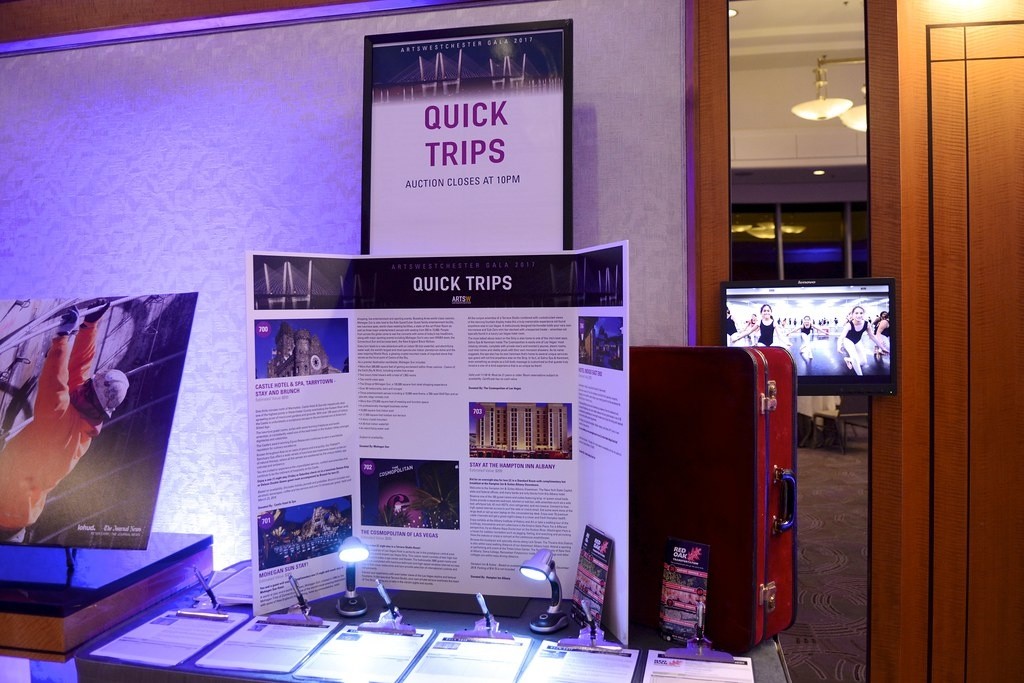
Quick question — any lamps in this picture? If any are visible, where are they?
[520,550,569,635]
[790,54,867,133]
[336,536,370,618]
[731,213,807,239]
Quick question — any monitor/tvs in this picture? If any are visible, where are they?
[722,276,898,396]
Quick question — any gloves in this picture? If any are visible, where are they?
[55,305,80,337]
[85,300,110,323]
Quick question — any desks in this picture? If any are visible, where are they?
[74,559,792,683]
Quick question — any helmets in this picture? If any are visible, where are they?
[91,368,130,418]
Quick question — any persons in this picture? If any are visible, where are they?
[727,303,890,376]
[0,297,130,544]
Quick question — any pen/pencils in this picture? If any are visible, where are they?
[697,600,706,645]
[287,576,309,611]
[194,566,217,607]
[376,579,397,619]
[476,593,491,627]
[581,599,597,639]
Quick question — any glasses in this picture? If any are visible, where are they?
[83,378,100,412]
